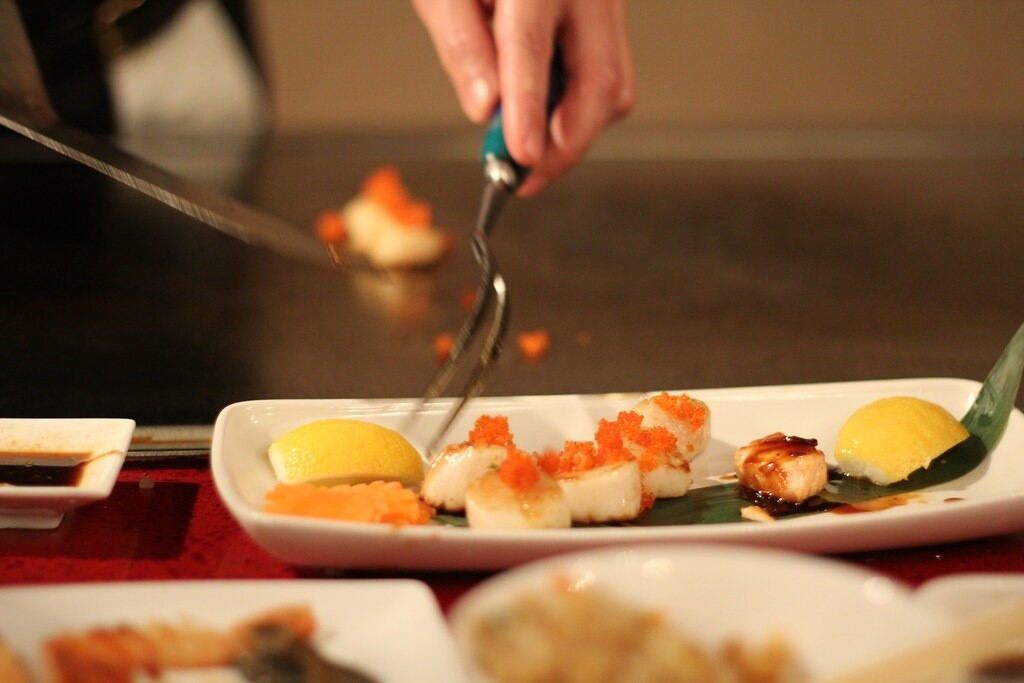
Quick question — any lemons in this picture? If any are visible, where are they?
[835,396,971,485]
[270,419,424,489]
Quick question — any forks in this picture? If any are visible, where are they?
[401,45,571,454]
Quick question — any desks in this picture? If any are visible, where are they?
[0,427,1024,622]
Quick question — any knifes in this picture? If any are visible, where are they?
[1,90,363,275]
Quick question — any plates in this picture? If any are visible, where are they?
[0,580,473,682]
[446,542,913,682]
[211,377,1024,566]
[913,570,1024,683]
[0,418,136,528]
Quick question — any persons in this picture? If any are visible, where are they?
[0,0,637,428]
